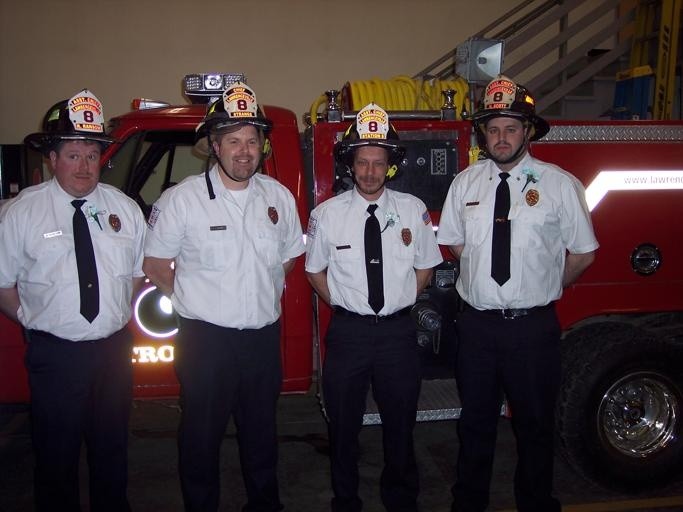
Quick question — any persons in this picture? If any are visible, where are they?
[437,73,600,511]
[0,88,149,511]
[304,102,443,511]
[142,83,305,512]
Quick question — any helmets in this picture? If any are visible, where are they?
[333,103,406,181]
[194,79,273,140]
[465,75,550,142]
[22,88,117,152]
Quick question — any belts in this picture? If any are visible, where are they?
[462,301,554,321]
[333,306,412,324]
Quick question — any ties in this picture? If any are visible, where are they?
[491,172,511,287]
[363,204,384,315]
[70,199,98,323]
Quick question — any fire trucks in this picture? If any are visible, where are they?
[0,1,681,493]
